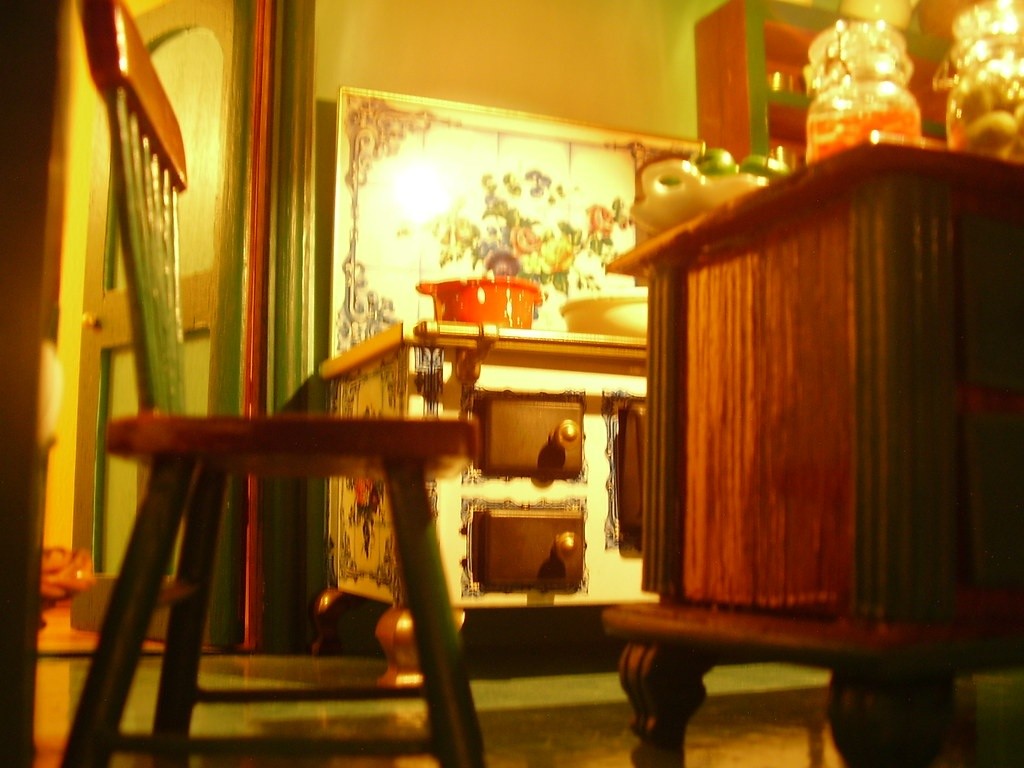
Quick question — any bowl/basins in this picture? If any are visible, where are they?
[417,276,542,329]
[559,293,649,337]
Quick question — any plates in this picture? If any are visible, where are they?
[630,159,769,237]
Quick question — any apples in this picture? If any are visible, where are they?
[662,148,791,185]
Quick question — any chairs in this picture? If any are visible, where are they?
[60,0,488,768]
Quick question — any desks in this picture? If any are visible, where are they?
[594,137,1024,768]
[310,318,648,678]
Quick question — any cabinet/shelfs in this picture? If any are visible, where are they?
[694,0,952,160]
